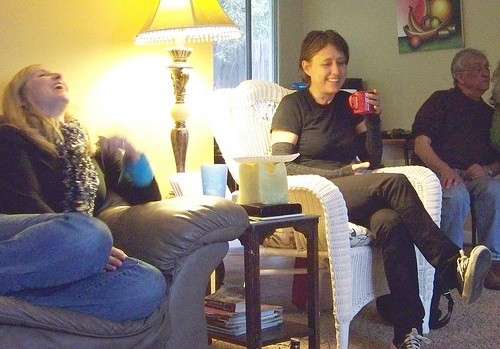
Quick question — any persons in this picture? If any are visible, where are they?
[269,27,491,349]
[0,63,167,322]
[410,47,500,290]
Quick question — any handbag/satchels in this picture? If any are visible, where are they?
[376,288,455,329]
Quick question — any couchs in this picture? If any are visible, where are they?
[0,184,249,349]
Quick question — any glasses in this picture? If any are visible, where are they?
[463,65,491,72]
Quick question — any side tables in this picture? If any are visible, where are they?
[204,215,322,348]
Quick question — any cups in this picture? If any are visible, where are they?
[348,91,376,115]
[200,163,228,197]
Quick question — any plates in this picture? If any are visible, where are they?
[233,153,301,165]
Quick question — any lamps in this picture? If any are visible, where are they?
[132,0,241,196]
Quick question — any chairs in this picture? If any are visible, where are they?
[404,136,476,248]
[211,79,443,349]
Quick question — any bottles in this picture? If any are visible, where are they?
[290,337,301,349]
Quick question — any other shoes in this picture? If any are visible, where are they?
[484,260,500,290]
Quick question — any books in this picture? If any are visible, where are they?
[205,282,285,337]
[168,170,234,205]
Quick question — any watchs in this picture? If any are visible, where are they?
[487,167,492,177]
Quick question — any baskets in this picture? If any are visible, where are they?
[233,153,300,164]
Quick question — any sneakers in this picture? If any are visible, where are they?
[390,322,431,349]
[455,245,492,305]
